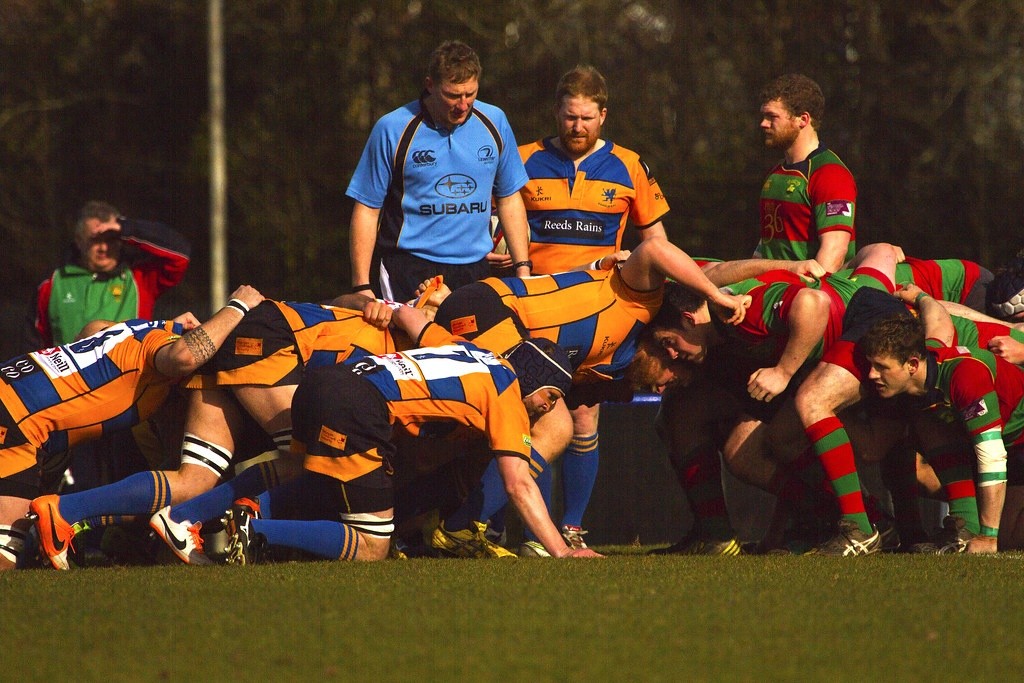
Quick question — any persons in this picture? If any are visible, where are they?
[24,203,191,349]
[345,41,533,300]
[748,74,859,275]
[490,64,671,559]
[0,232,1024,572]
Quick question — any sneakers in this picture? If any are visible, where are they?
[220,505,258,566]
[879,514,929,553]
[150,505,215,567]
[23,492,79,569]
[431,521,519,560]
[560,524,589,550]
[507,533,551,558]
[232,496,264,519]
[647,524,744,557]
[803,520,885,557]
[100,523,154,561]
[751,531,796,556]
[68,517,108,568]
[909,516,977,555]
[391,506,440,558]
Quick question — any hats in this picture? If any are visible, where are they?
[498,334,574,403]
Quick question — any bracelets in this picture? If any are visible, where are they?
[184,327,217,366]
[352,284,371,292]
[513,261,532,271]
[227,299,251,316]
